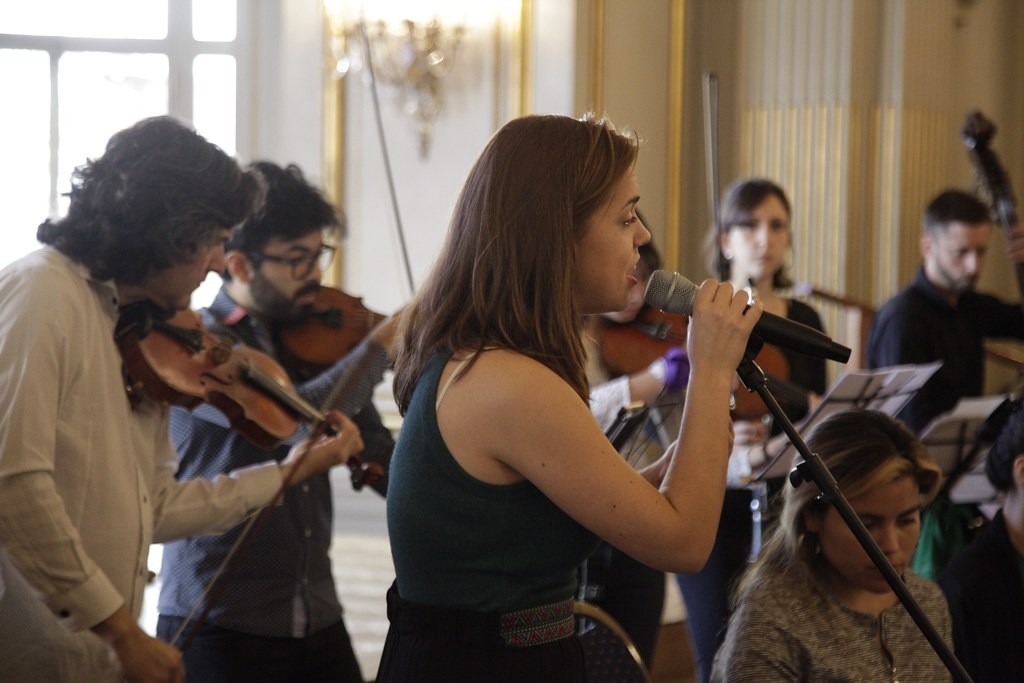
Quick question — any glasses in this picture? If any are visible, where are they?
[226,242,337,278]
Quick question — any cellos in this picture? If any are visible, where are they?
[959,107,1024,307]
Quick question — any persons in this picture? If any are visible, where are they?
[0,117,408,683]
[578,177,1024,682]
[377,115,828,683]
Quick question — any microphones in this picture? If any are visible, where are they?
[644,270,852,364]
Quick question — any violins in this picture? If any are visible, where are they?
[271,284,390,378]
[113,304,386,493]
[598,303,822,421]
